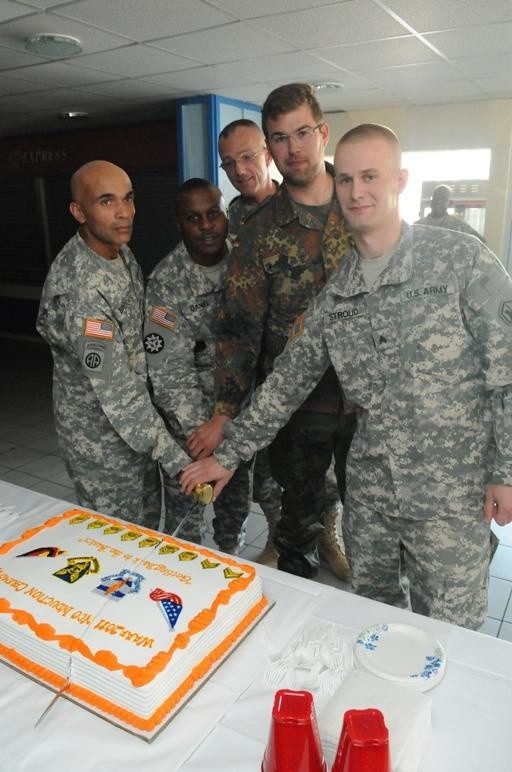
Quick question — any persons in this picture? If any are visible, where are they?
[411,185,485,242]
[142,176,253,557]
[176,124,512,633]
[186,82,356,579]
[217,117,349,580]
[34,156,196,530]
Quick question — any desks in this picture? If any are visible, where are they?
[0,478,512,772]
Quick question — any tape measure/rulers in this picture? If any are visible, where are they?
[171,484,213,537]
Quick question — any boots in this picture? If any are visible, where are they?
[314,507,355,582]
[263,503,283,544]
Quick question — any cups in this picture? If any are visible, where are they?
[261,689,392,772]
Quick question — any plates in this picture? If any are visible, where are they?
[355,623,447,693]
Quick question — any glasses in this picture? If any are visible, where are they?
[218,149,264,172]
[264,122,325,149]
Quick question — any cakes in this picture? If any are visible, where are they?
[0,507,269,734]
